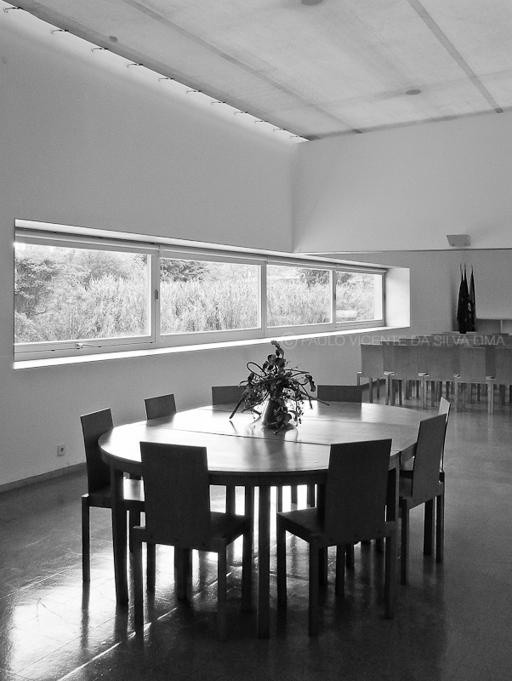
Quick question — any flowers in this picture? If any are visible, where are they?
[229,340,330,434]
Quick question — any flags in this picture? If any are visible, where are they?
[456,271,476,335]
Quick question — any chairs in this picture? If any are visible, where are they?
[354,331,509,410]
